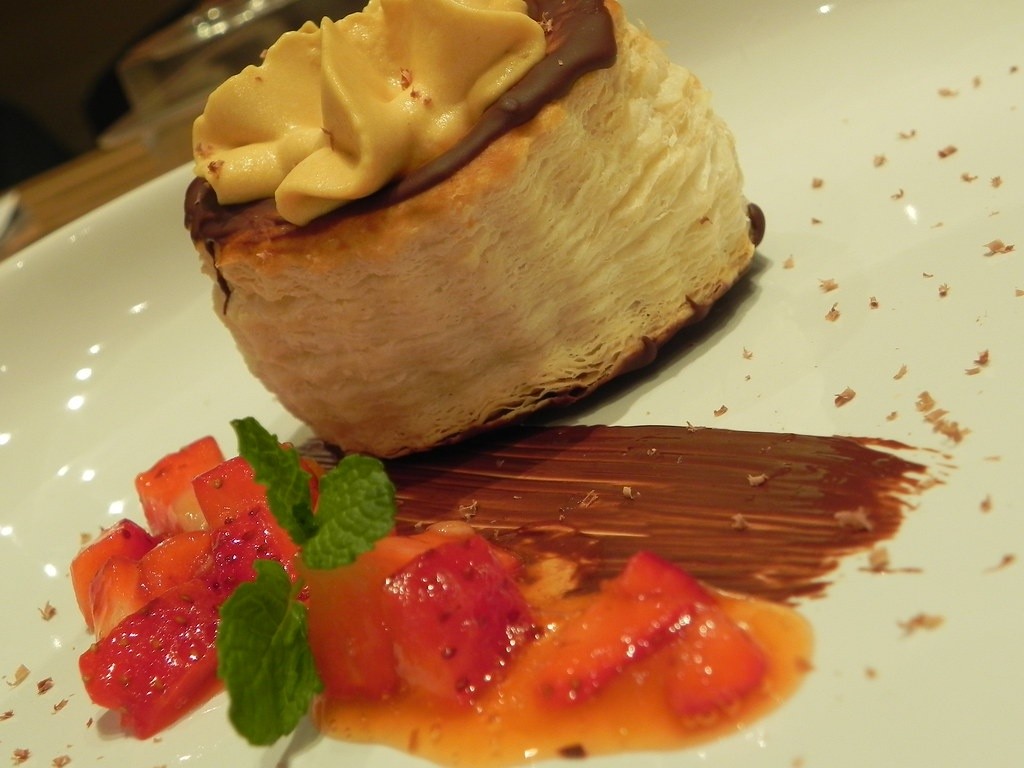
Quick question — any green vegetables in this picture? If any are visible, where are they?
[215,417,397,745]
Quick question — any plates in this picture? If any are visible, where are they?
[0,3,1024,763]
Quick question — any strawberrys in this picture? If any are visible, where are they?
[68,438,764,741]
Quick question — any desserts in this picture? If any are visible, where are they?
[178,0,764,460]
